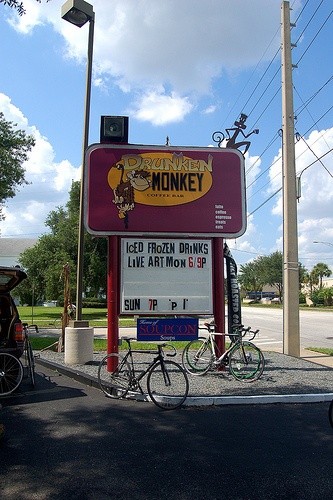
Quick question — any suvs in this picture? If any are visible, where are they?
[0,266,28,381]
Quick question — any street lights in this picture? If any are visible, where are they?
[61,0,95,328]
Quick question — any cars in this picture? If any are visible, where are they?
[248,300,260,304]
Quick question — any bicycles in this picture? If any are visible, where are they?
[97,335,189,410]
[22,323,39,385]
[181,323,266,384]
[0,352,24,396]
